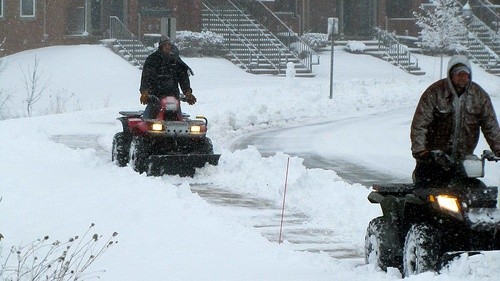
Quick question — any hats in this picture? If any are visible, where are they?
[159,35,173,45]
[451,66,471,76]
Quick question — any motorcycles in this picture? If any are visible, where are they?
[363,150,500,278]
[111,94,221,178]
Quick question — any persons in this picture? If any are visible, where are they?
[139,36,196,120]
[410,55,500,188]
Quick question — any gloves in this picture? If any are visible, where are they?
[413,151,435,163]
[140,90,149,105]
[186,90,196,104]
